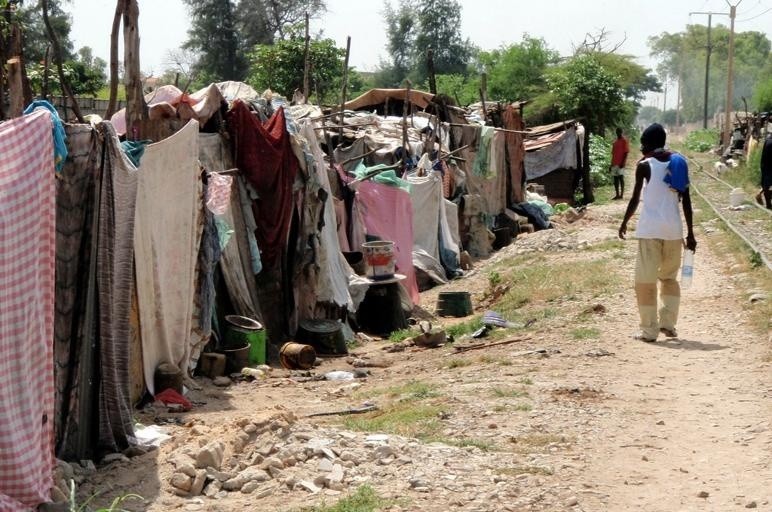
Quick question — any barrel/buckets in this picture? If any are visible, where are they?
[730,192,746,206]
[434,291,476,320]
[277,341,317,370]
[230,326,266,366]
[363,240,396,281]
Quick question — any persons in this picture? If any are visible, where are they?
[755,132,772,209]
[619,122,698,342]
[607,127,630,201]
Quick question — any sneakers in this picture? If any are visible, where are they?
[632,326,677,342]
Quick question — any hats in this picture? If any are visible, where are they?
[640,123,666,146]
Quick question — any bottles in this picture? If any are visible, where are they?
[681,247,694,290]
